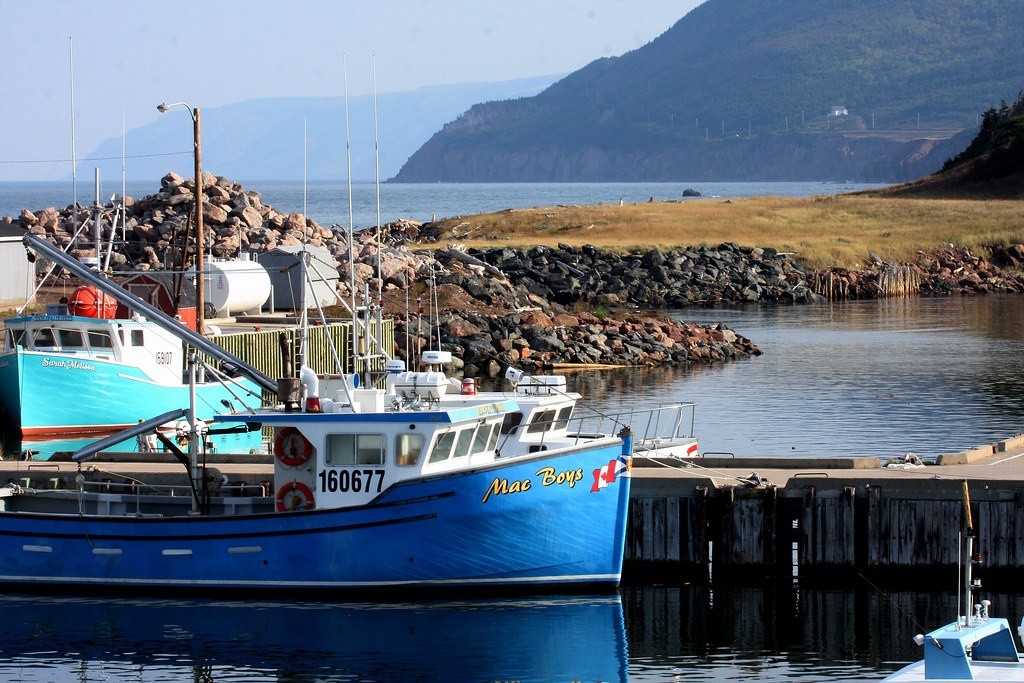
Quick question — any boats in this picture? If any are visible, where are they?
[877,478,1024,683]
[291,52,703,461]
[0,585,634,683]
[1,245,636,595]
[0,33,263,464]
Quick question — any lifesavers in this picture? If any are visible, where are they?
[276,482,314,512]
[274,427,313,466]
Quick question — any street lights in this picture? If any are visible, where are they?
[155,99,207,336]
